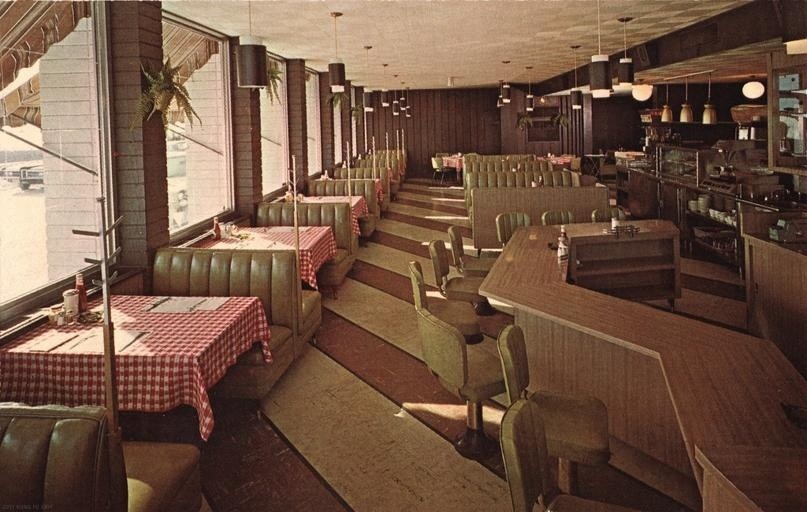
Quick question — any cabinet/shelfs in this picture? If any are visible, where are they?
[615,152,806,279]
[761,40,807,179]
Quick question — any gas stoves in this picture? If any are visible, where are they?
[699,171,769,194]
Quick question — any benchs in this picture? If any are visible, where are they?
[1,402,204,509]
[463,153,574,226]
[308,150,407,246]
[151,247,323,420]
[255,202,356,298]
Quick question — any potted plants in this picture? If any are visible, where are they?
[121,54,202,133]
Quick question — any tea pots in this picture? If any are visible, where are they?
[777,136,792,156]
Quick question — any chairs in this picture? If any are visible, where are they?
[496,211,531,249]
[428,239,488,302]
[448,226,498,275]
[542,210,574,224]
[408,258,484,345]
[414,302,510,458]
[591,208,626,221]
[499,398,631,510]
[498,323,613,496]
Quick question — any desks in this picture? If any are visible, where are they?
[738,209,803,378]
[2,292,273,440]
[479,221,804,510]
[585,153,608,179]
[199,226,337,287]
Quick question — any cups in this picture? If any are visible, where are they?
[629,160,651,171]
[703,234,736,252]
[601,217,620,233]
[747,187,806,206]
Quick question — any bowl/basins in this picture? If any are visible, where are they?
[686,193,738,228]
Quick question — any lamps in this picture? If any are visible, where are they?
[236,1,413,122]
[492,0,765,131]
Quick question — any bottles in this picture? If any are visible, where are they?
[556,224,569,275]
[212,216,239,241]
[531,175,544,188]
[644,125,682,148]
[320,170,332,182]
[510,163,521,172]
[284,184,303,203]
[733,126,756,142]
[46,272,88,328]
